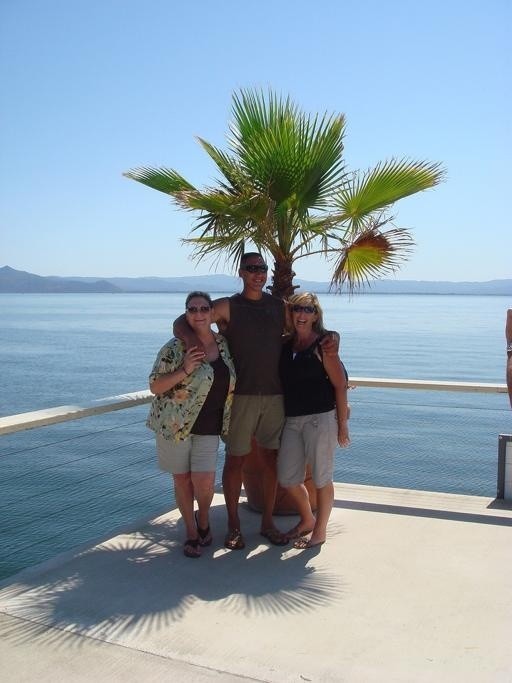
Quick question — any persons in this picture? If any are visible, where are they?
[145,292,237,558]
[173,253,340,549]
[276,292,350,549]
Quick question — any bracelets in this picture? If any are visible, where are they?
[181,365,190,376]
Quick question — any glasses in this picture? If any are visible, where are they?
[244,264,268,273]
[187,307,209,313]
[292,305,315,312]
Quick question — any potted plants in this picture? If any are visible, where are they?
[124,83,449,520]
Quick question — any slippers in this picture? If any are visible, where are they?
[260,527,289,546]
[224,528,245,550]
[184,510,212,558]
[287,527,325,548]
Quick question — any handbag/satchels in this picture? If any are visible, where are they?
[309,361,349,398]
